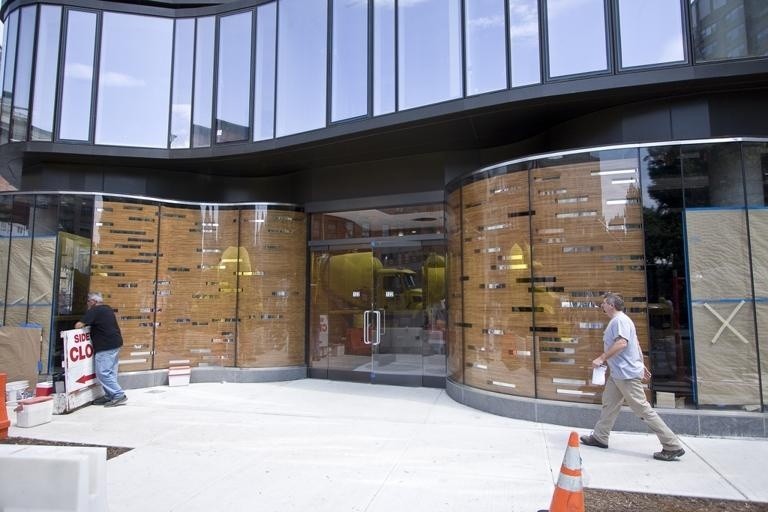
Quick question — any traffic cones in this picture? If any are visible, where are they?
[548,431,585,512]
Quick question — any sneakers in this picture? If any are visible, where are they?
[653,447,685,460]
[579,433,609,448]
[103,394,128,407]
[93,395,110,405]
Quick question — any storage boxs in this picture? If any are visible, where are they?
[167,367,192,387]
[13,395,55,428]
[35,381,54,396]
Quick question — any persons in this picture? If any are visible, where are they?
[73,291,127,407]
[577,291,684,462]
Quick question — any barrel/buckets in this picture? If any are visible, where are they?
[5,381,30,401]
